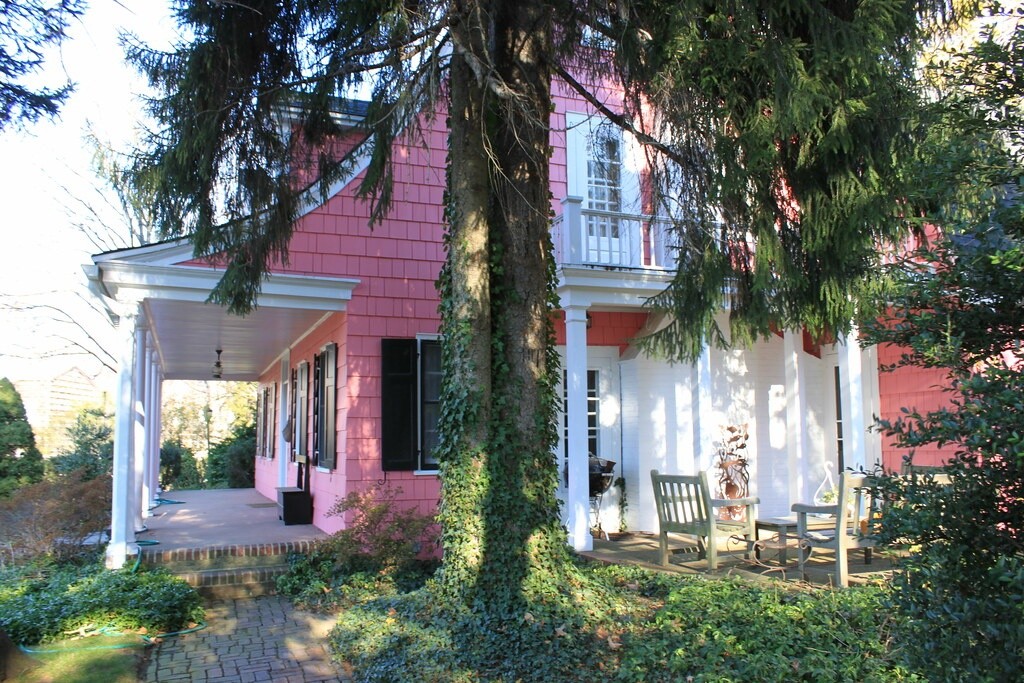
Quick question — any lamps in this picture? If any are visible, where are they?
[210,350,226,378]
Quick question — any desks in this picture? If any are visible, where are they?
[749,513,872,571]
[275,486,313,526]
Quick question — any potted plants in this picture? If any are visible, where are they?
[811,485,856,519]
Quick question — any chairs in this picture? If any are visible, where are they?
[647,468,762,576]
[789,470,886,591]
